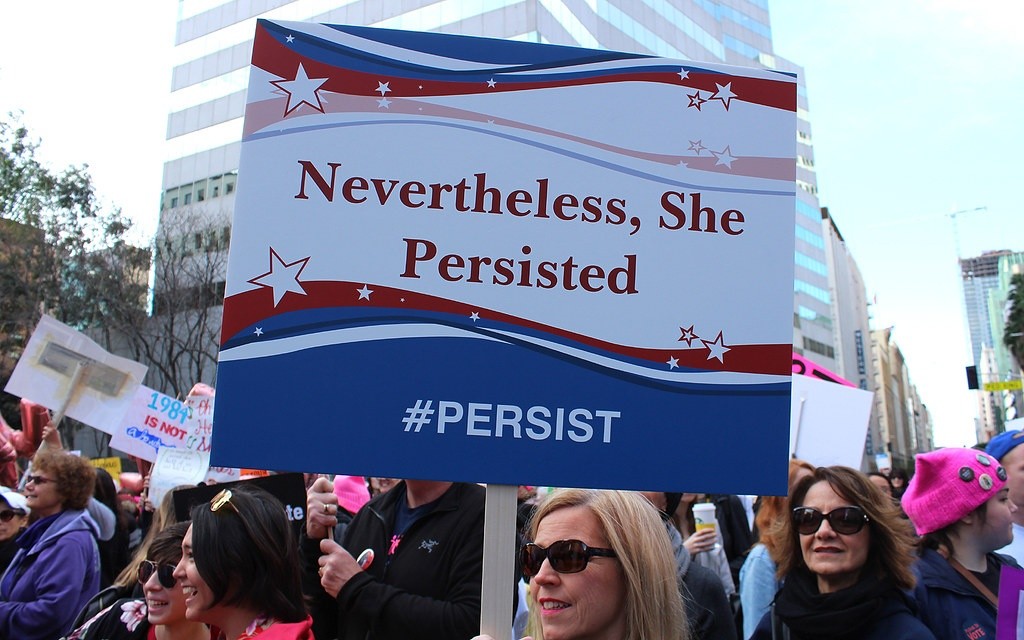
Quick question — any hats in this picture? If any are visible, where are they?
[901,447,1008,536]
[0,492,32,516]
[0,486,12,493]
[333,474,370,513]
[985,430,1024,462]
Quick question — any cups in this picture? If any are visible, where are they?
[692,502,716,550]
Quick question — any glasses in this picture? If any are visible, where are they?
[0,510,26,522]
[210,489,241,517]
[791,506,870,535]
[136,559,178,589]
[28,475,59,485]
[659,509,672,521]
[519,539,621,577]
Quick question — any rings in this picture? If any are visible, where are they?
[324,504,329,514]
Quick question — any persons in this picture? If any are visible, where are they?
[0,429,1023,639]
